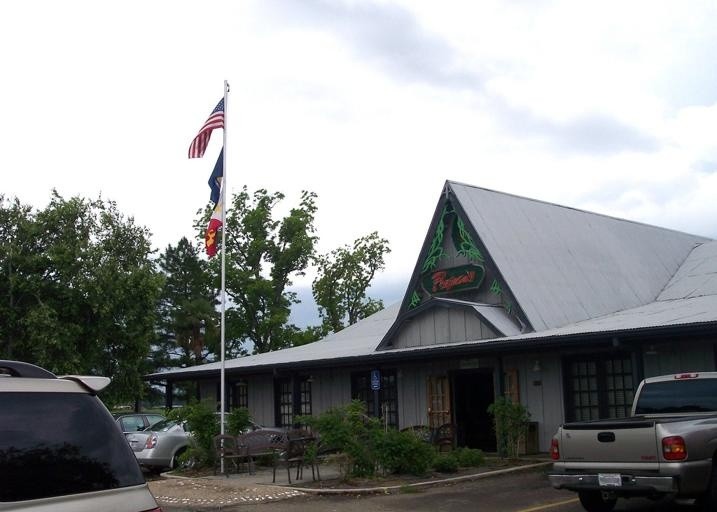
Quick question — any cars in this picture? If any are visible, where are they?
[111,412,165,434]
[0,358,164,512]
[123,412,290,472]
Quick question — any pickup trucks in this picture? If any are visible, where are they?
[546,370,717,512]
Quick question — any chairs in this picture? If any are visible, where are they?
[432,424,457,452]
[214,428,320,484]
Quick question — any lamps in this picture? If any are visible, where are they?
[237,371,248,386]
[532,349,543,372]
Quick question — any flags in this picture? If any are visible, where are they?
[187,94,224,158]
[205,145,224,257]
